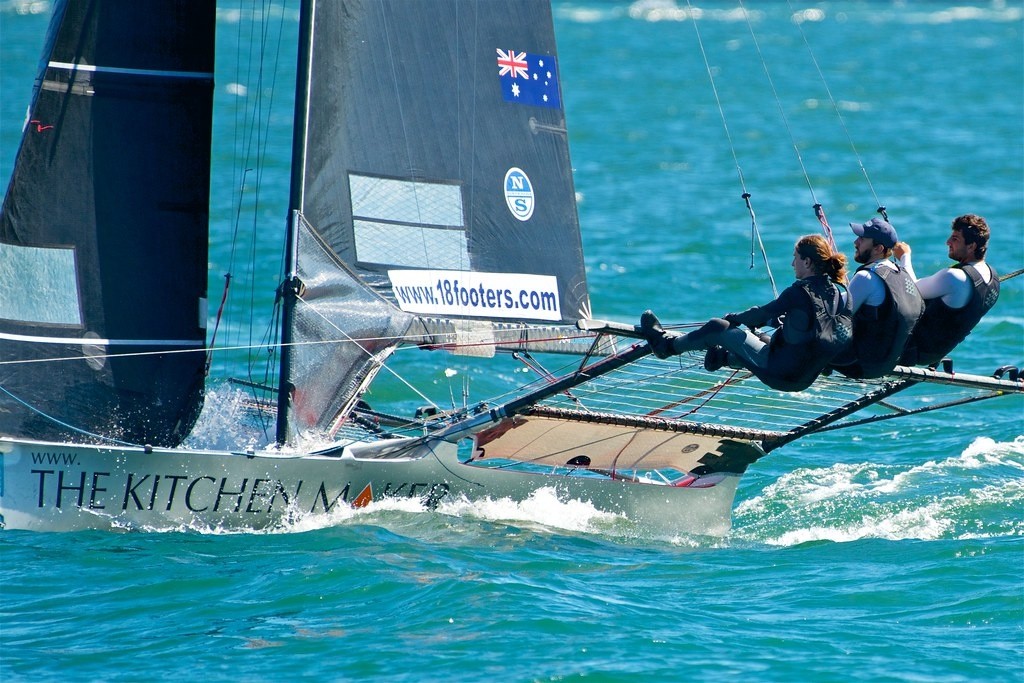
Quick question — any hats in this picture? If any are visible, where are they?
[849,218,897,248]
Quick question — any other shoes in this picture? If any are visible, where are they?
[704,345,728,372]
[641,310,681,359]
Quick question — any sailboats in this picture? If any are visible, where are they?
[0,2,1024,545]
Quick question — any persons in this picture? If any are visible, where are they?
[749,217,925,380]
[892,214,1000,369]
[641,234,851,392]
